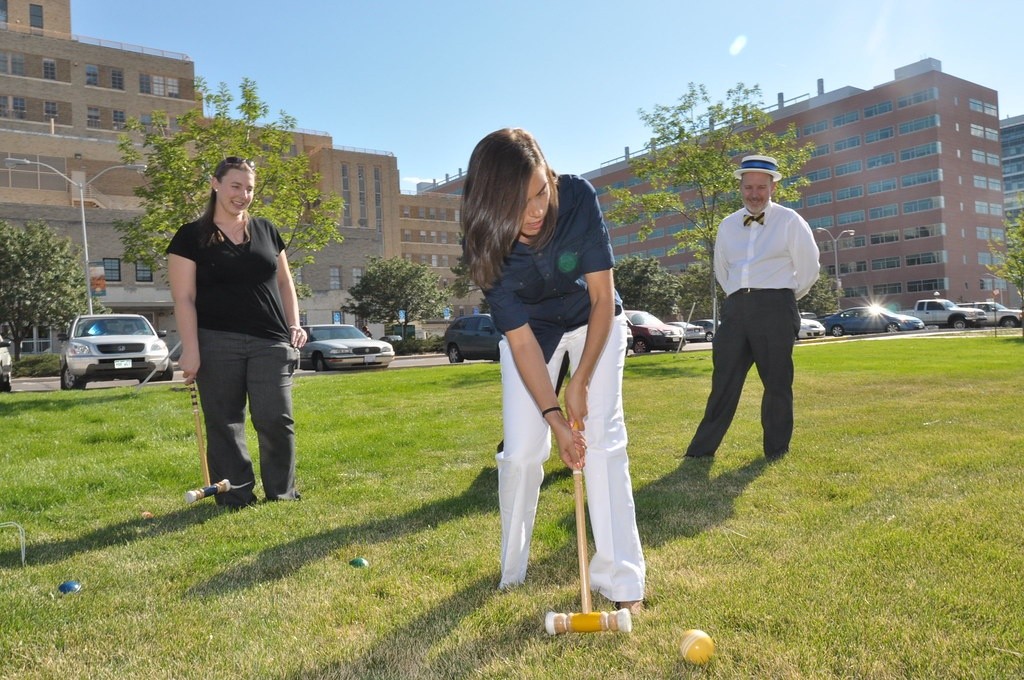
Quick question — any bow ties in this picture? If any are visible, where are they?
[742,212,766,227]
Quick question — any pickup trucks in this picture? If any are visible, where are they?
[895,297,988,329]
[956,301,1024,330]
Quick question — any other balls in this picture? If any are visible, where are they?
[58,580,83,594]
[349,557,369,568]
[679,629,714,666]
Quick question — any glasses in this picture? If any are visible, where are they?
[225,156,256,170]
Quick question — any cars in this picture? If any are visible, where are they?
[443,312,503,363]
[795,312,826,340]
[379,335,403,343]
[624,309,687,354]
[664,321,707,343]
[57,313,174,390]
[689,319,722,342]
[0,335,14,393]
[299,324,396,372]
[818,306,926,337]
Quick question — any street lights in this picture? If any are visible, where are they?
[3,157,148,317]
[815,226,856,309]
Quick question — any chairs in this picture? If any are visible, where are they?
[124,323,137,334]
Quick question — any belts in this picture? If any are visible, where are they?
[733,288,768,295]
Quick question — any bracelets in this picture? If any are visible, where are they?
[542,407,563,418]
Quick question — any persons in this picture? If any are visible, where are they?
[164,157,307,514]
[683,155,821,458]
[460,128,646,616]
[361,326,372,338]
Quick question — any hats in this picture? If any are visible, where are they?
[733,155,783,183]
